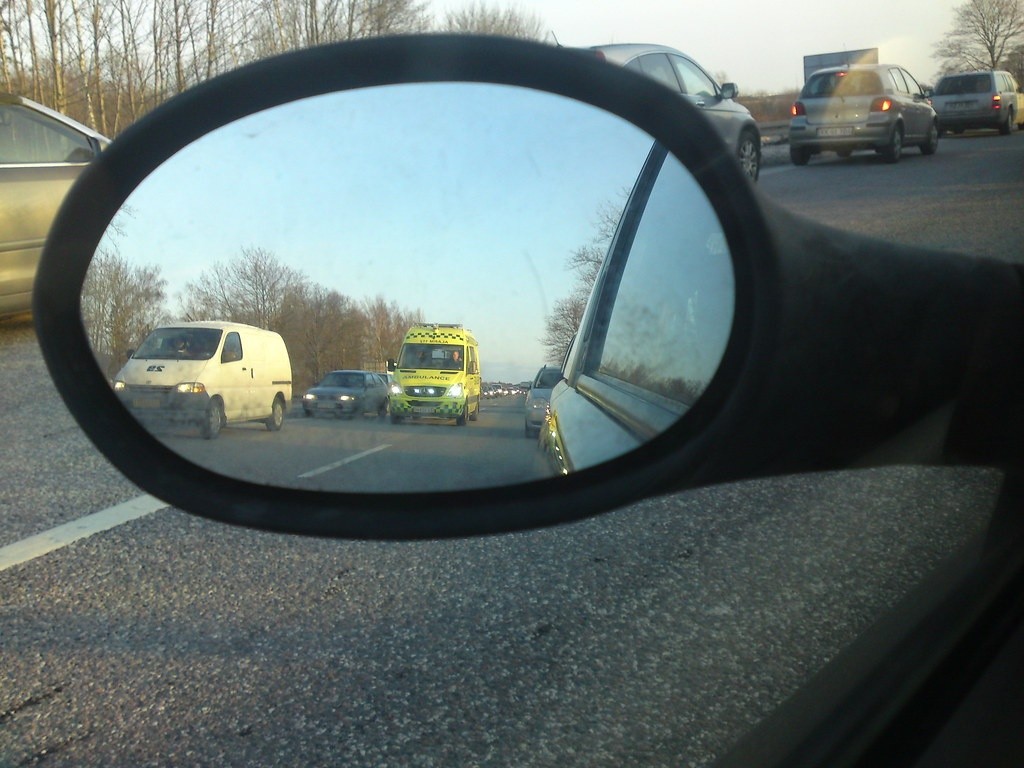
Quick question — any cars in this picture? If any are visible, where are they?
[589,43,769,188]
[479,384,527,399]
[788,63,941,160]
[1,94,122,325]
[301,369,389,422]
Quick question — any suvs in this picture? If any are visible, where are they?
[928,71,1024,131]
[524,362,566,439]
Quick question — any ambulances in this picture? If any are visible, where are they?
[383,322,485,426]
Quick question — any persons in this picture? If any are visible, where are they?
[447,351,462,368]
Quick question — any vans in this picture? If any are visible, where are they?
[107,320,293,438]
